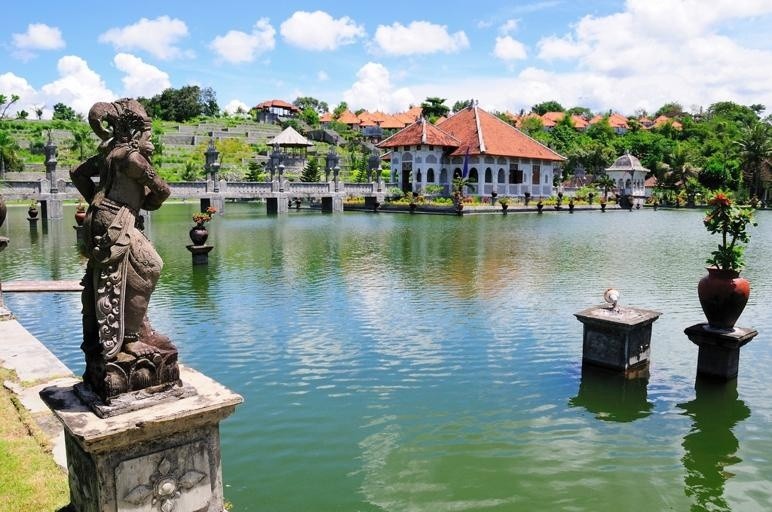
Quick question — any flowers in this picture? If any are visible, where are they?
[697,189,757,269]
[191,205,217,226]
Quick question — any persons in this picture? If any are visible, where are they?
[68,97,165,358]
[557,182,564,193]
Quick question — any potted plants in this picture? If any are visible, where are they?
[26,201,39,218]
[74,195,86,227]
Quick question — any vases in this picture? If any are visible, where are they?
[490,191,606,210]
[696,266,752,331]
[407,191,418,210]
[188,224,208,246]
